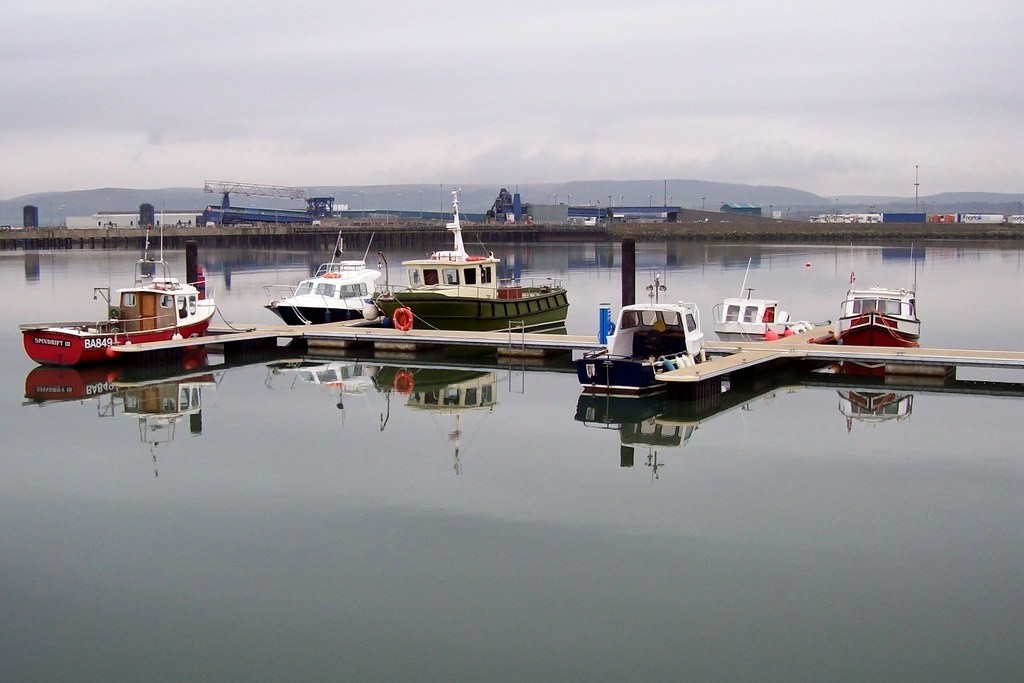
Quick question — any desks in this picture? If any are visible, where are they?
[635,330,685,337]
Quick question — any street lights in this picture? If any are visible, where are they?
[668,195,672,207]
[786,208,789,221]
[920,200,925,213]
[608,194,612,207]
[835,198,839,220]
[553,193,559,206]
[648,194,653,208]
[620,196,623,206]
[769,205,772,220]
[701,197,706,211]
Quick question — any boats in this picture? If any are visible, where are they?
[21,345,217,478]
[263,327,574,477]
[712,256,815,343]
[833,358,913,435]
[833,242,921,350]
[18,208,217,368]
[575,275,711,393]
[363,187,571,335]
[264,230,390,326]
[574,388,682,485]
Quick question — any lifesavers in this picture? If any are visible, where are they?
[393,307,414,331]
[393,372,414,395]
[323,273,342,279]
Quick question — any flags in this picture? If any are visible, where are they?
[334,234,344,258]
[849,271,856,284]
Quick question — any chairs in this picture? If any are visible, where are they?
[645,329,663,350]
